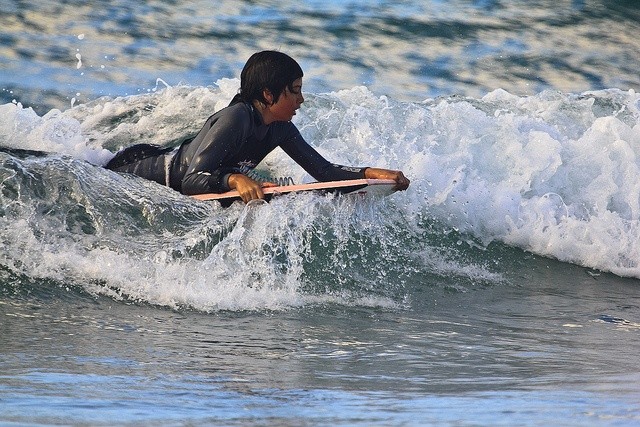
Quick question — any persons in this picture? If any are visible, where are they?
[105,51,410,205]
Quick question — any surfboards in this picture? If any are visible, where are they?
[189,176,397,209]
